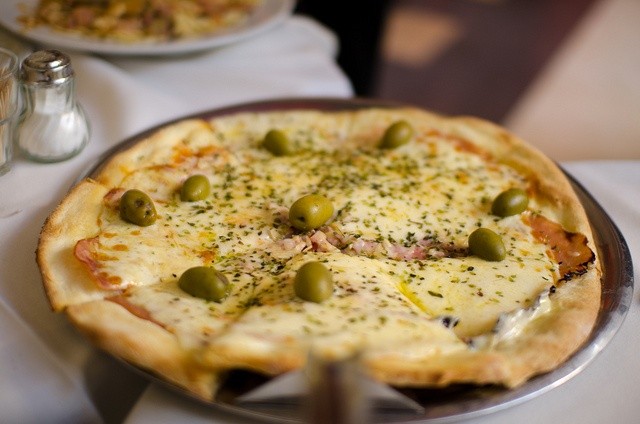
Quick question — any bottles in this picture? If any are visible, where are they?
[13,48,93,164]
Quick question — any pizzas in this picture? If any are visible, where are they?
[36,104,602,402]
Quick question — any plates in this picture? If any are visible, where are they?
[0,2,297,55]
[64,98,635,422]
